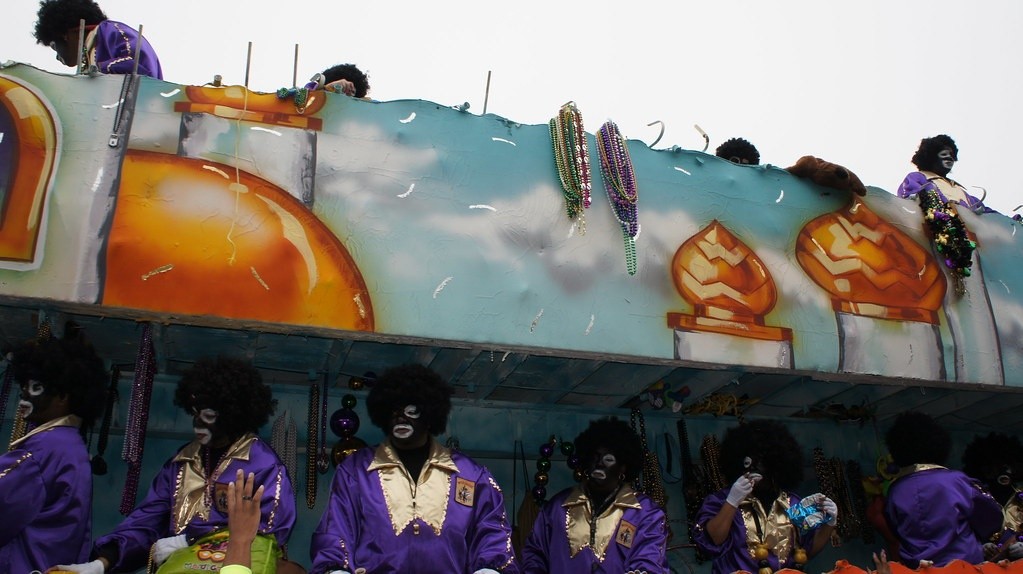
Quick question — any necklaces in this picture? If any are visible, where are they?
[915,188,976,298]
[270,370,329,560]
[147,543,154,574]
[406,462,421,534]
[632,408,674,540]
[0,320,159,517]
[585,481,622,546]
[687,430,724,564]
[204,447,227,506]
[810,444,864,548]
[277,88,307,114]
[596,120,641,274]
[549,106,592,235]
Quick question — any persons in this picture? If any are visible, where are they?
[851,410,1023,567]
[717,138,760,167]
[42,350,297,574]
[0,336,94,574]
[898,136,1023,224]
[308,362,519,574]
[521,417,671,574]
[34,0,164,80]
[305,63,370,98]
[691,418,838,574]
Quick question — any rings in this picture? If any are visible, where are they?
[242,496,252,500]
